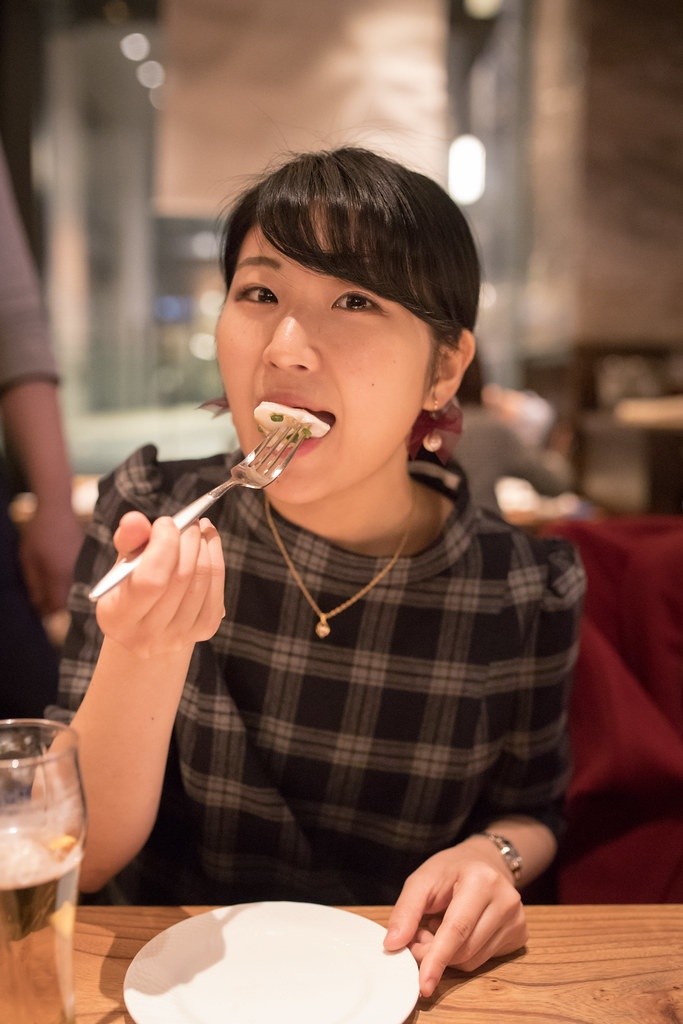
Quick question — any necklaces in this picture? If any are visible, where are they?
[265,481,418,637]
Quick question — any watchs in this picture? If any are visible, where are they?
[477,831,522,889]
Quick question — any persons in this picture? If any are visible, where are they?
[33,147,586,998]
[0,151,85,721]
[450,351,573,520]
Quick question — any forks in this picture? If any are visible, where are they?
[89,424,313,600]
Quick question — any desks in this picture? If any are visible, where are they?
[70,906,683,1024]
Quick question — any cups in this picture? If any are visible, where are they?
[0,720,87,1024]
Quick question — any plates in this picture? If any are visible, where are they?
[123,901,420,1024]
